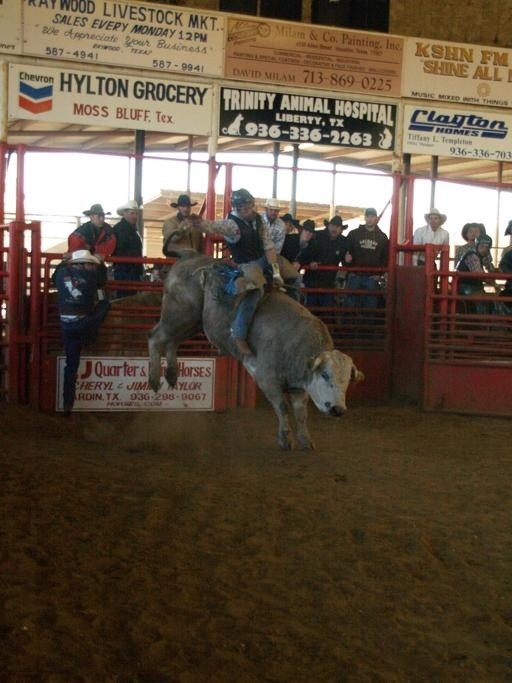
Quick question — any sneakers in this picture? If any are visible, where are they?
[231,335,253,357]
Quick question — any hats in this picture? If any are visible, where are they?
[296,220,319,233]
[365,207,376,217]
[259,198,288,211]
[116,199,144,216]
[230,187,256,208]
[280,214,300,226]
[424,207,448,227]
[67,249,102,266]
[462,223,486,242]
[323,215,349,231]
[82,203,112,217]
[169,194,198,208]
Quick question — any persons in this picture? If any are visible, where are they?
[52,249,112,419]
[68,202,117,255]
[186,187,286,356]
[145,256,165,281]
[111,199,146,297]
[260,198,510,338]
[161,193,205,281]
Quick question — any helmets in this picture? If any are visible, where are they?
[474,234,493,248]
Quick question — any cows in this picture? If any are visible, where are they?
[146,231,366,451]
[39,290,169,356]
